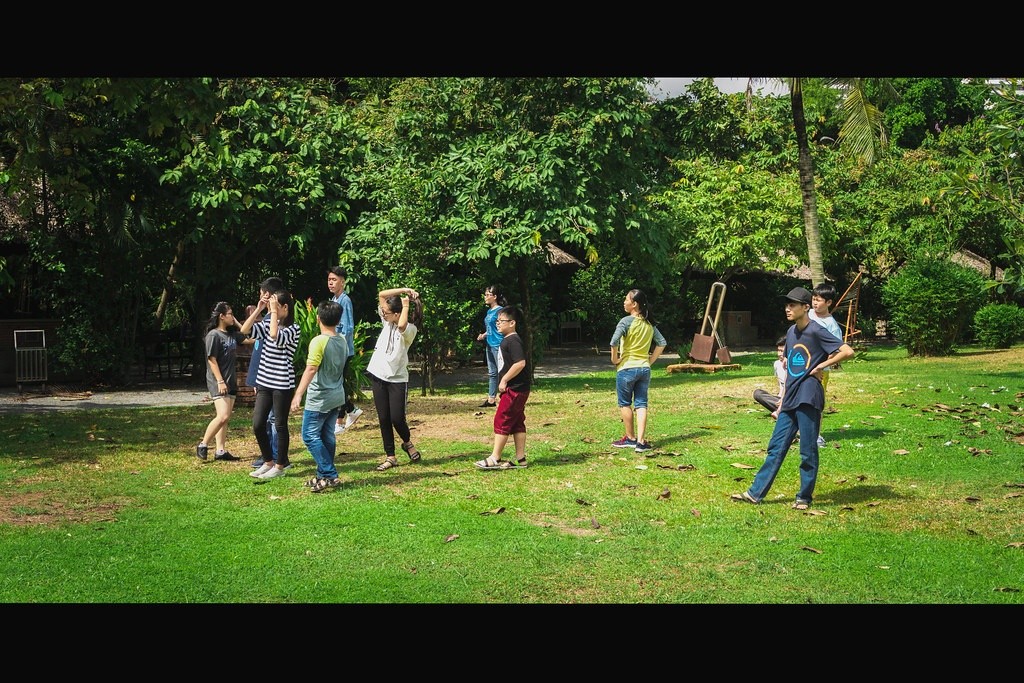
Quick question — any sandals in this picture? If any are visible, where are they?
[500,457,527,469]
[310,478,340,492]
[376,457,398,471]
[401,442,420,461]
[304,478,322,487]
[473,456,501,469]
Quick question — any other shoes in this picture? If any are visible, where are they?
[477,399,496,409]
[817,436,825,446]
[251,457,265,467]
[214,452,240,461]
[284,465,290,469]
[770,411,778,419]
[197,446,207,460]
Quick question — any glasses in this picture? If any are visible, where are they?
[382,309,395,315]
[224,312,234,316]
[496,319,512,323]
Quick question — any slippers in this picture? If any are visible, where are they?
[730,493,756,504]
[791,501,808,510]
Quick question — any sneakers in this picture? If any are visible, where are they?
[612,435,637,447]
[249,463,271,477]
[345,408,363,428]
[258,466,285,478]
[334,424,346,433]
[635,441,652,452]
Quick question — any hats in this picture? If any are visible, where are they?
[779,287,812,306]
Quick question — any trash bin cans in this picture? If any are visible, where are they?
[13,330,48,394]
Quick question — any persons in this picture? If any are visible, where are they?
[610,289,667,452]
[196,302,255,462]
[474,306,530,470]
[290,301,349,492]
[477,285,507,407]
[808,283,842,445]
[731,287,854,510]
[754,335,786,412]
[240,277,301,479]
[366,288,423,470]
[327,266,363,434]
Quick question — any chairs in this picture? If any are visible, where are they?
[550,313,583,346]
[140,326,196,379]
